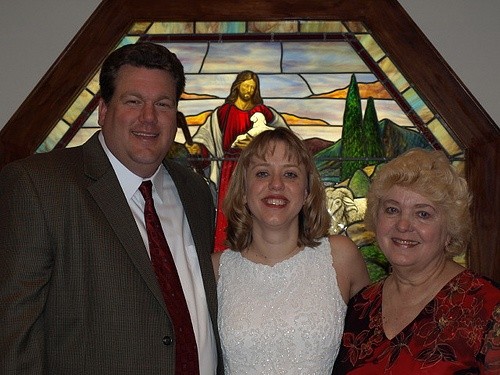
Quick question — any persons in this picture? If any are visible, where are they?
[209,127,373,375]
[0,41,226,375]
[331,146,500,375]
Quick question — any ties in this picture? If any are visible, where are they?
[139,179,201,375]
